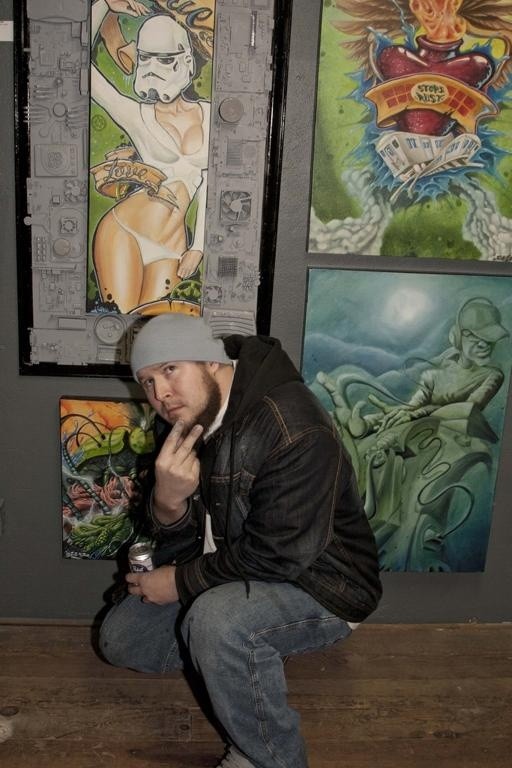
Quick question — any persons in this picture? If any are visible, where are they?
[96,309,387,768]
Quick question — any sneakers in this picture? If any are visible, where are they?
[216,745,258,768]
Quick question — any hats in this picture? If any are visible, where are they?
[459,303,510,343]
[130,312,233,385]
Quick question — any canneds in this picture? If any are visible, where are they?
[128,542,155,586]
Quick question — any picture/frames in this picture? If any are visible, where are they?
[12,0,294,378]
